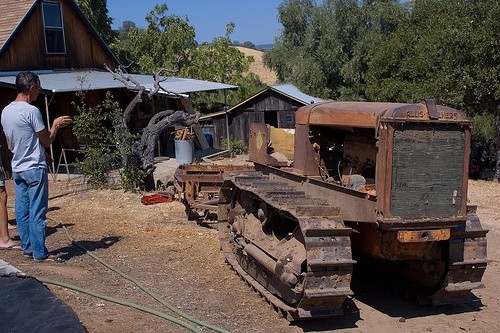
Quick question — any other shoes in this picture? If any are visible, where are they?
[23,252,33,257]
[33,254,65,262]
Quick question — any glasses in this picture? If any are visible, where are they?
[36,86,42,92]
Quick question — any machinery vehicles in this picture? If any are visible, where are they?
[175,95,494,324]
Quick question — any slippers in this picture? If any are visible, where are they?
[0,241,22,250]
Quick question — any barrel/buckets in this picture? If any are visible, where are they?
[204,133,213,148]
[174,139,193,163]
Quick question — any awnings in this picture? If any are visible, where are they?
[0,71,238,182]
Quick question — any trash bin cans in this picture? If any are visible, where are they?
[174,138,196,164]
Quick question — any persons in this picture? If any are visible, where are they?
[0,126,22,250]
[0,72,72,262]
